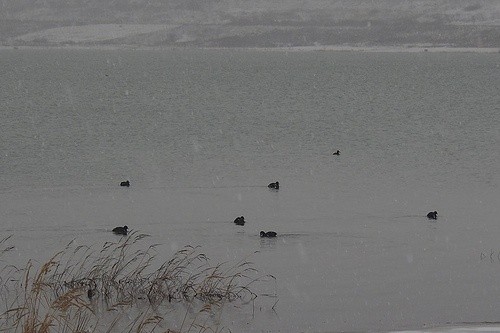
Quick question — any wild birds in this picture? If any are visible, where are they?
[112,226,129,235]
[234,216,245,225]
[260,231,277,237]
[427,211,437,220]
[120,180,129,186]
[268,181,279,189]
[332,150,340,155]
